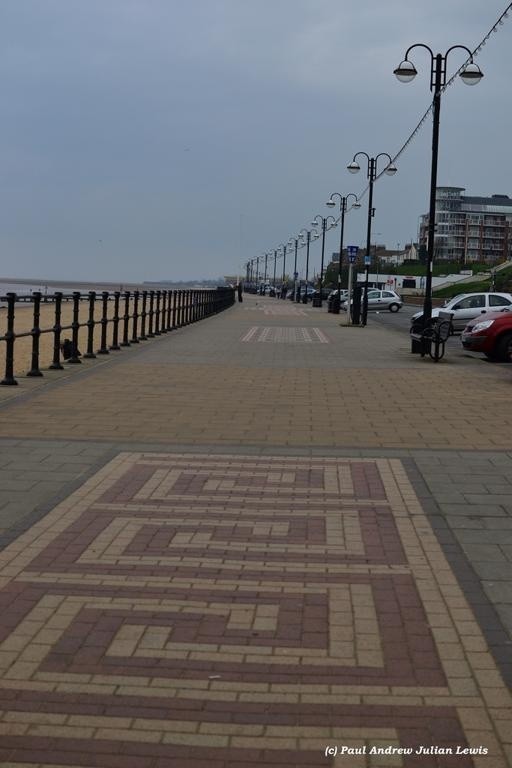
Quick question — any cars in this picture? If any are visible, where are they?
[460,312,512,363]
[411,291,512,335]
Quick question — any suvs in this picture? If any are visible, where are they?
[328,286,403,314]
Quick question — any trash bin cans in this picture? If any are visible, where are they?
[410,310,428,353]
[328,290,340,314]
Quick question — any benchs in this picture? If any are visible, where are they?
[409,312,454,362]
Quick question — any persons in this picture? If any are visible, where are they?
[282,285,288,300]
[233,282,243,302]
[275,285,281,299]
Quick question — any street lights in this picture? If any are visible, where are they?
[395,43,482,353]
[245,215,338,298]
[346,151,396,325]
[327,192,361,315]
